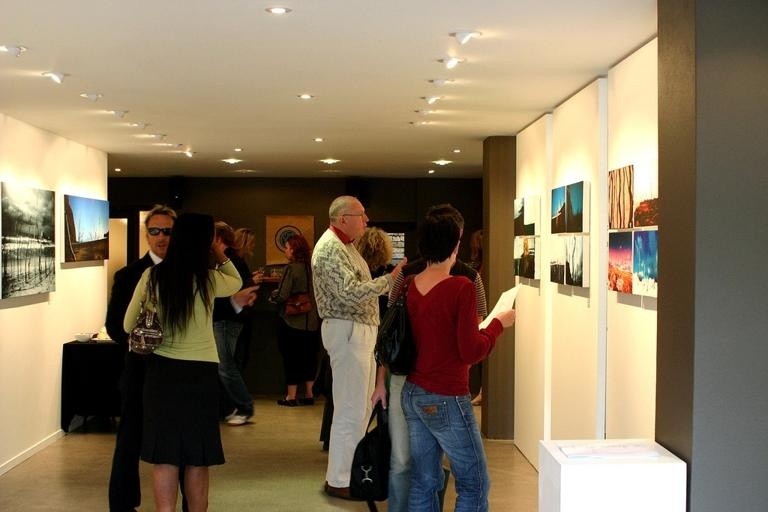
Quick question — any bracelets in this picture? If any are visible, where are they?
[218,257,230,268]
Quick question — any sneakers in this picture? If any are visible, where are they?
[297,397,315,406]
[223,408,254,426]
[277,397,299,407]
[324,481,353,501]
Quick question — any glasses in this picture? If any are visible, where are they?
[146,227,173,236]
[342,211,366,219]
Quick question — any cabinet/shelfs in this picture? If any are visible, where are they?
[536,439,687,512]
[61,339,123,436]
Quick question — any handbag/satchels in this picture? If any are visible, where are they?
[127,301,163,357]
[373,295,417,376]
[349,415,390,503]
[283,292,313,315]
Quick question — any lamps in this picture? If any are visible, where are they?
[439,58,463,71]
[421,95,443,105]
[151,134,165,144]
[184,151,197,159]
[433,158,454,166]
[450,29,483,45]
[136,122,148,131]
[174,144,182,149]
[3,45,26,59]
[113,108,130,119]
[41,63,66,86]
[432,79,446,88]
[80,89,103,105]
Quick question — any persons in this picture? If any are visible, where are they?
[394,214,515,512]
[470,230,484,405]
[371,203,486,511]
[268,235,319,407]
[309,195,406,501]
[122,213,242,511]
[235,227,263,375]
[105,205,260,511]
[518,239,534,280]
[211,220,253,426]
[355,228,394,429]
